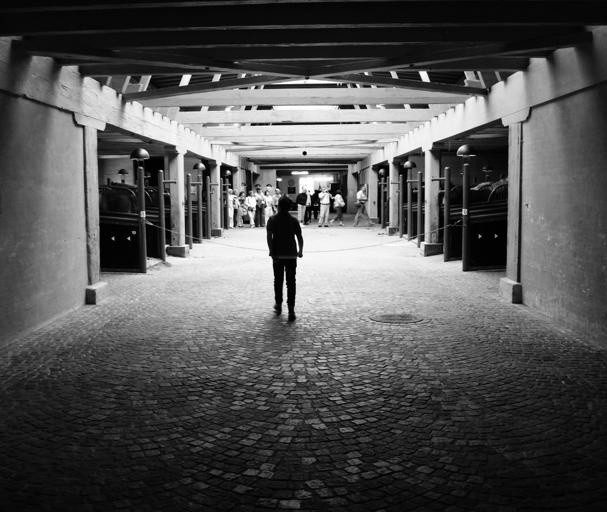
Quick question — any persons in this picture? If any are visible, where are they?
[267,193,304,321]
[226,182,375,227]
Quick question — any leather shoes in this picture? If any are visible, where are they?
[300,219,375,228]
[288,314,296,322]
[229,223,267,228]
[274,305,282,312]
